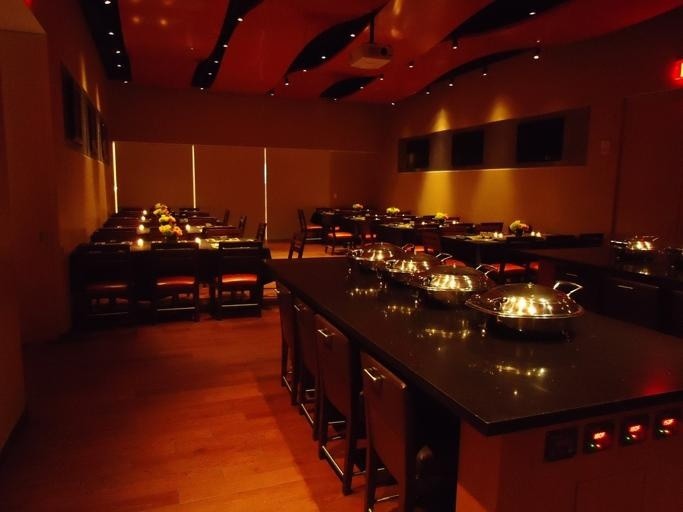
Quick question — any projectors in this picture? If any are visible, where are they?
[349,41,392,71]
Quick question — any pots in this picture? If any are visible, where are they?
[615,260,655,279]
[346,241,586,342]
[469,342,562,382]
[610,234,660,259]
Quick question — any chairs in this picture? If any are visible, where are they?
[479,230,681,338]
[285,200,503,260]
[276,283,295,406]
[359,347,458,508]
[295,298,319,440]
[315,314,385,495]
[90,206,265,241]
[148,242,198,324]
[75,243,137,329]
[209,241,265,321]
